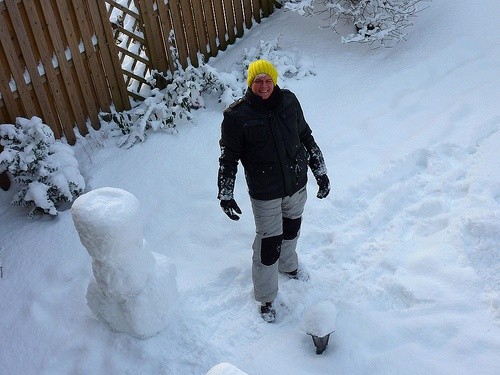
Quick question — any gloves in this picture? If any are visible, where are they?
[315,174,331,199]
[220,199,242,221]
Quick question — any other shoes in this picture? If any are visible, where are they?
[261,302,275,322]
[277,269,310,282]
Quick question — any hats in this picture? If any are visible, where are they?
[247,59,278,88]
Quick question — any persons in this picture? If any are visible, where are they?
[217,60,332,324]
